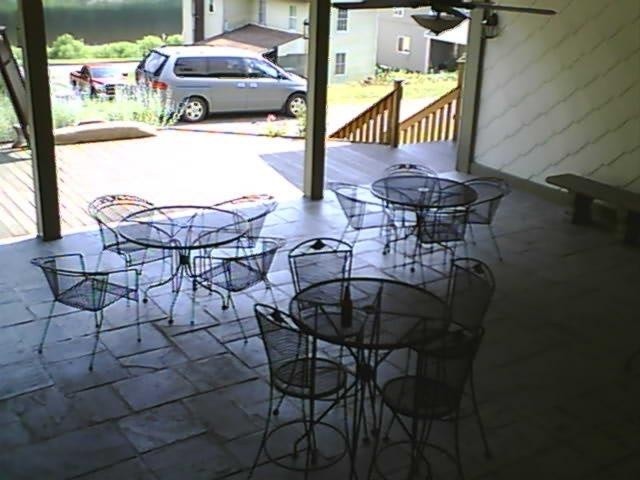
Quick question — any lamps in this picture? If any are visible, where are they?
[410,7,466,36]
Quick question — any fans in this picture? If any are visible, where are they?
[331,0,557,19]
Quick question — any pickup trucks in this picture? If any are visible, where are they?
[70,62,131,101]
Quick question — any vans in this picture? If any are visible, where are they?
[135,46,308,122]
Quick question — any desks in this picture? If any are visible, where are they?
[544,173,639,242]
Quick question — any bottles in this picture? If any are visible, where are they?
[341,286,353,329]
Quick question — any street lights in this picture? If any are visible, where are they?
[304,18,310,78]
